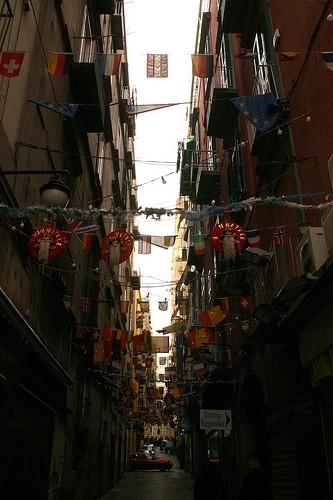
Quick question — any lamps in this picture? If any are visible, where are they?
[3,169,72,208]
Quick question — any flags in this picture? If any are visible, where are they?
[96,52,122,76]
[0,50,25,77]
[47,50,74,76]
[30,95,288,432]
[146,53,169,78]
[190,54,215,78]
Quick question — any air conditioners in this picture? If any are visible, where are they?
[294,226,329,277]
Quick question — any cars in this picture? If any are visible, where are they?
[139,444,155,457]
[127,452,174,472]
